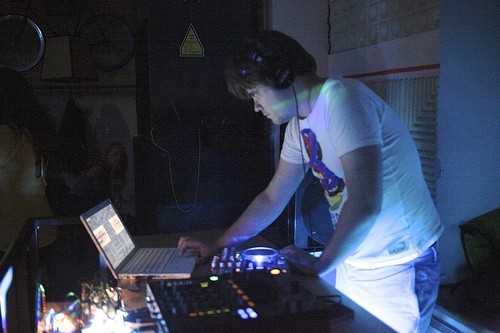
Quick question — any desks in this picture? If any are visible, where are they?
[99,229,396,333]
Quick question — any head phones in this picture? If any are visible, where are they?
[248,49,294,88]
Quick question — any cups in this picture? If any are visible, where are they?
[119,274,149,311]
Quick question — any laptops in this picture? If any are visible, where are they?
[79,198,198,279]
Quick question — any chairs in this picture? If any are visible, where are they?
[40,153,77,217]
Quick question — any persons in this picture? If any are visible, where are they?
[177,30,442,333]
